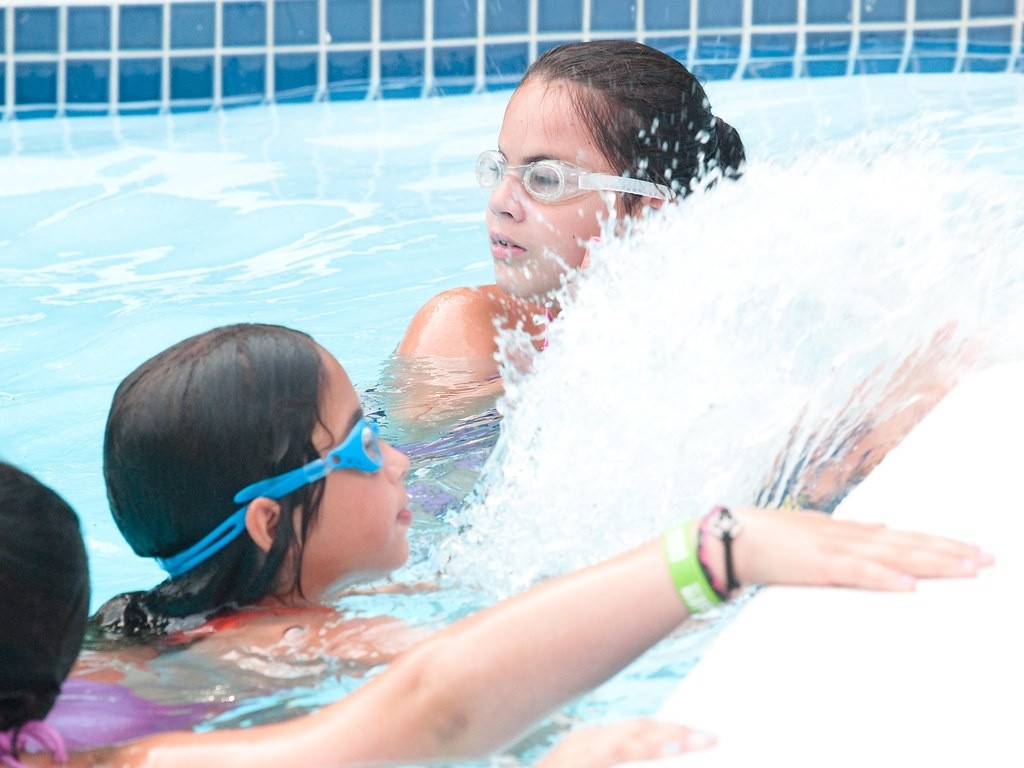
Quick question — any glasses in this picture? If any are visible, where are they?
[233,419,383,504]
[475,149,676,205]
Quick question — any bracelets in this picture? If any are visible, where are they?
[671,506,740,614]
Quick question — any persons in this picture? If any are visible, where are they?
[398,39,746,356]
[101,321,413,599]
[1,463,995,768]
